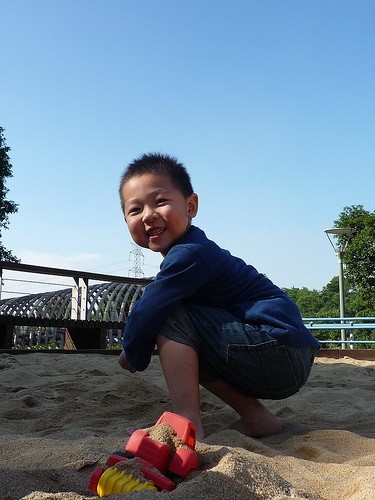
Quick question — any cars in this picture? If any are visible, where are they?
[16,328,61,347]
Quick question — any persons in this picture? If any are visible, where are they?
[117,155,320,442]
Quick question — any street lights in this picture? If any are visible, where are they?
[324,227,357,350]
